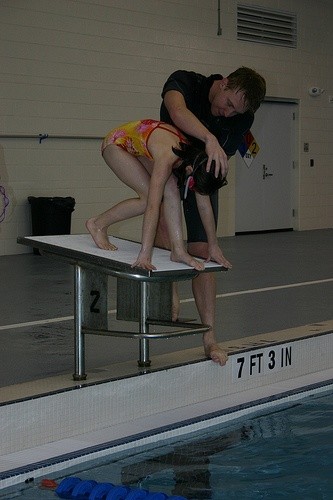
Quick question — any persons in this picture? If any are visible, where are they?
[86,118,235,272]
[157,64,267,367]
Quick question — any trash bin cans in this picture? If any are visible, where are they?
[26,195,76,255]
